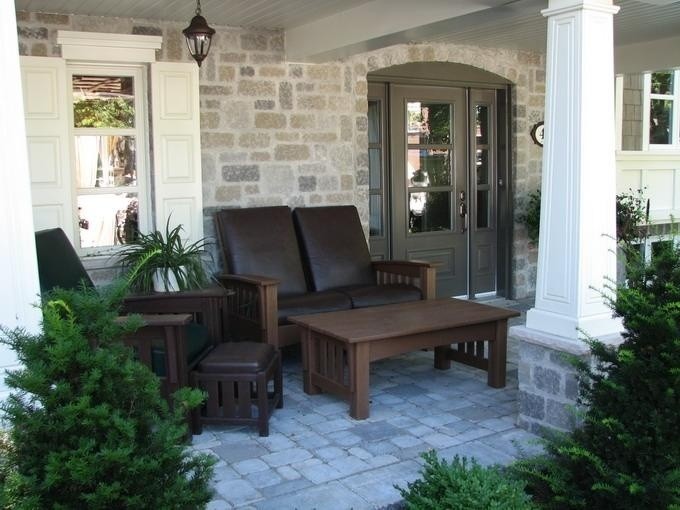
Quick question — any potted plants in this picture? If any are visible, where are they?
[102,211,224,295]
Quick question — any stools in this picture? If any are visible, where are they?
[192,340,281,436]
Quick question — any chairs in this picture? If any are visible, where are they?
[32,226,232,434]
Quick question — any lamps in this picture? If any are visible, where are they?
[182,1,219,70]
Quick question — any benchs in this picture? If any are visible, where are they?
[212,205,439,353]
